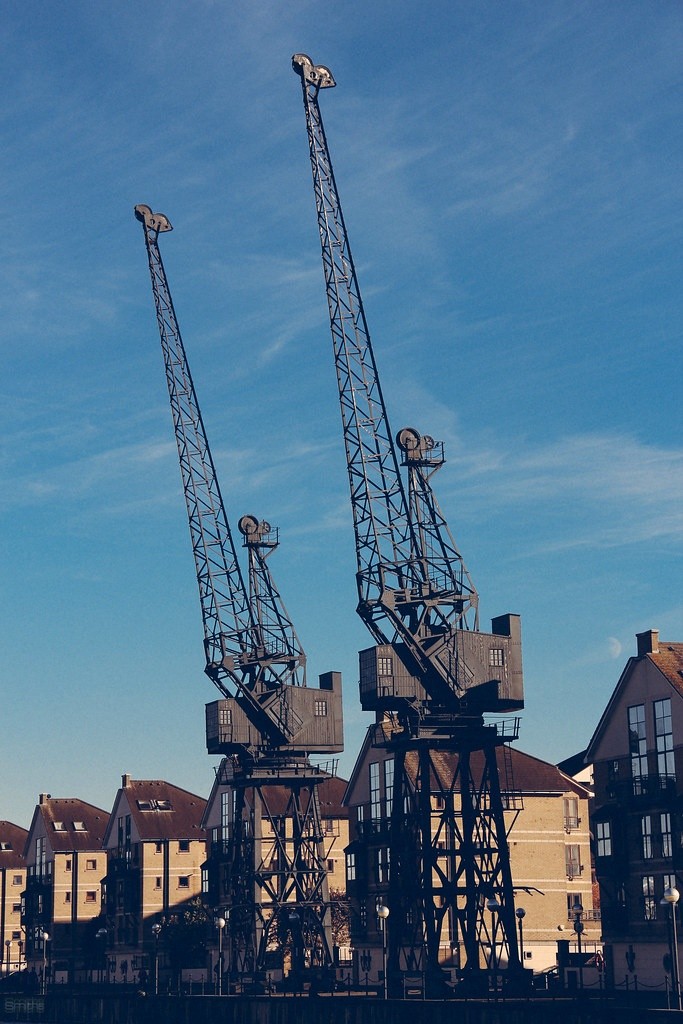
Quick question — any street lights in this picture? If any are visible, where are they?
[17,940,24,976]
[485,898,501,1001]
[213,918,227,997]
[40,931,51,996]
[376,904,391,999]
[664,888,683,1012]
[573,905,584,998]
[149,924,162,995]
[5,939,10,977]
[96,929,107,996]
[514,907,526,969]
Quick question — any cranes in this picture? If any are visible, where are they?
[286,50,529,713]
[137,206,351,754]
